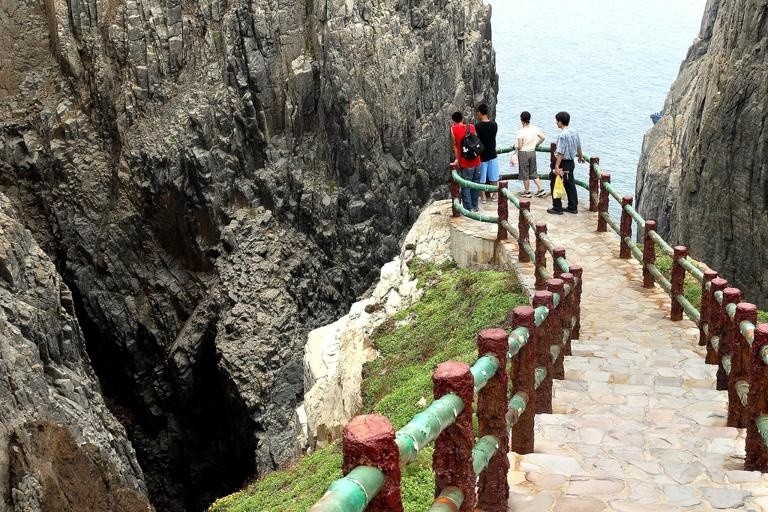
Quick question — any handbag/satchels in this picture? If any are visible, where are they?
[509,147,519,168]
[460,132,484,161]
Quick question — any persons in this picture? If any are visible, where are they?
[450,111,484,211]
[509,111,547,198]
[546,111,585,215]
[474,104,500,205]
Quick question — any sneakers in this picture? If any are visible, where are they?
[519,191,532,198]
[480,195,488,204]
[563,207,577,214]
[466,206,479,212]
[491,195,498,202]
[547,208,563,215]
[535,189,547,197]
